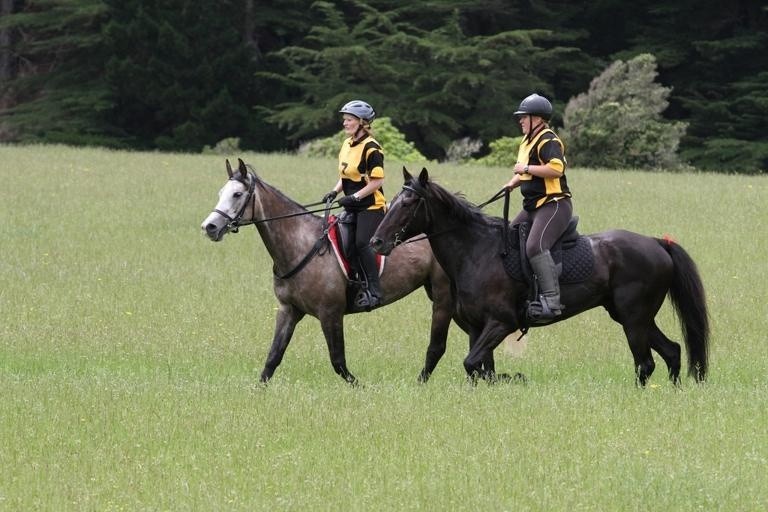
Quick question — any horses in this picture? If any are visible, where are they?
[200,157,496,394]
[366,165,711,390]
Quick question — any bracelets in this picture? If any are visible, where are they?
[353,194,360,202]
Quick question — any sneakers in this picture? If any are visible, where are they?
[361,296,378,308]
[530,305,562,317]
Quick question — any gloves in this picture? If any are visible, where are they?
[337,195,356,206]
[323,191,337,204]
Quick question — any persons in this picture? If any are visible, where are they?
[321,100,387,307]
[504,93,573,317]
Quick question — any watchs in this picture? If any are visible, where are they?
[524,164,529,174]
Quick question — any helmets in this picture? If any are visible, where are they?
[339,100,376,124]
[512,92,553,120]
